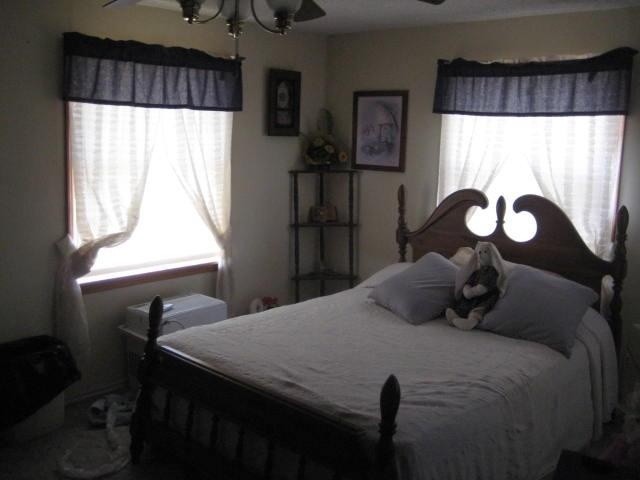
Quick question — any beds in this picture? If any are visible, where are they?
[126,185,629,480]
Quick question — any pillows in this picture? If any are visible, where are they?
[371,244,598,356]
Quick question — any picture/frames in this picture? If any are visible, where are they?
[351,90,408,171]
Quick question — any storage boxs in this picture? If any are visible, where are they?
[118,294,227,390]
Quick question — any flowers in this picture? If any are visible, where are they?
[300,107,348,171]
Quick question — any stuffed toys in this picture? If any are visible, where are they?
[445,241,508,331]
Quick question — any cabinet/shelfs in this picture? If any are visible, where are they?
[290,169,359,303]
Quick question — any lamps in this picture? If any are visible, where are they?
[176,1,305,38]
